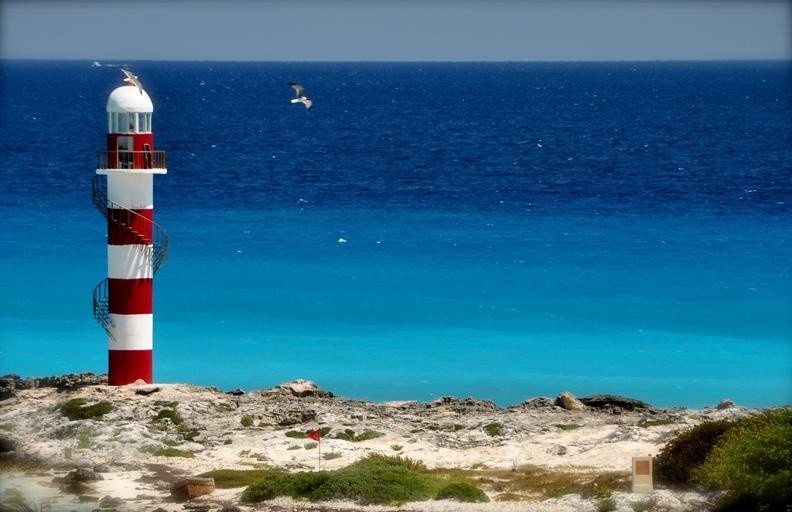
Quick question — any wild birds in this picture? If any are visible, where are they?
[120,68,142,95]
[288,81,305,99]
[291,95,312,109]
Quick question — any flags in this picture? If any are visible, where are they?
[308,429,321,441]
